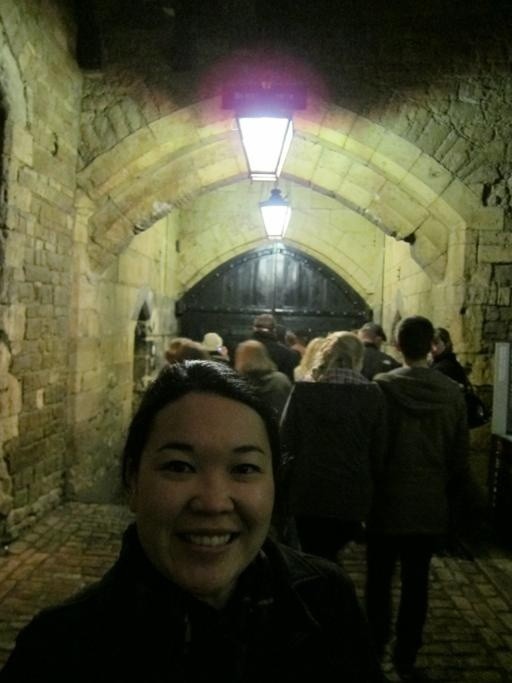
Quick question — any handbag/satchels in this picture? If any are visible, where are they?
[466,392,490,429]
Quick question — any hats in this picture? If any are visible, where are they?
[202,332,224,352]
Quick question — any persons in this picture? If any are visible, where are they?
[372,315,470,674]
[0,359,389,681]
[278,331,386,562]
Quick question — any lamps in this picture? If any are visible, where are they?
[234,98,306,240]
